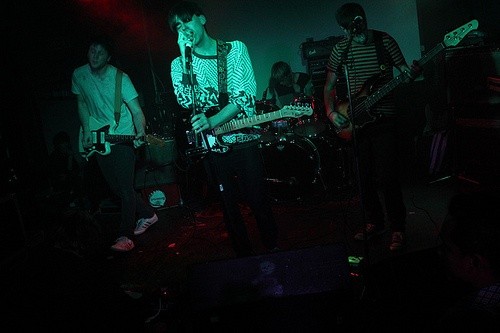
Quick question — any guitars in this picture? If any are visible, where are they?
[180,101,313,154]
[78,116,162,158]
[334,20,478,140]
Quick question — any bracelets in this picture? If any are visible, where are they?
[328,110,334,118]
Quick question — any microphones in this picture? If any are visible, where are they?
[185,32,193,62]
[350,16,364,27]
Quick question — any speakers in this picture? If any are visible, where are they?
[441,193,500,274]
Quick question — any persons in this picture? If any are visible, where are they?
[70,37,159,252]
[167,2,280,259]
[324,3,417,255]
[272,61,315,99]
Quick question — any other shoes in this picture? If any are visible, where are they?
[355,224,385,240]
[266,241,281,252]
[389,231,406,250]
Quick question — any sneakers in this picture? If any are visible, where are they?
[111,236,134,251]
[134,212,158,235]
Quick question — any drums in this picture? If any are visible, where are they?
[250,136,322,196]
[245,100,279,133]
[145,132,177,163]
[289,94,331,138]
[272,117,294,142]
